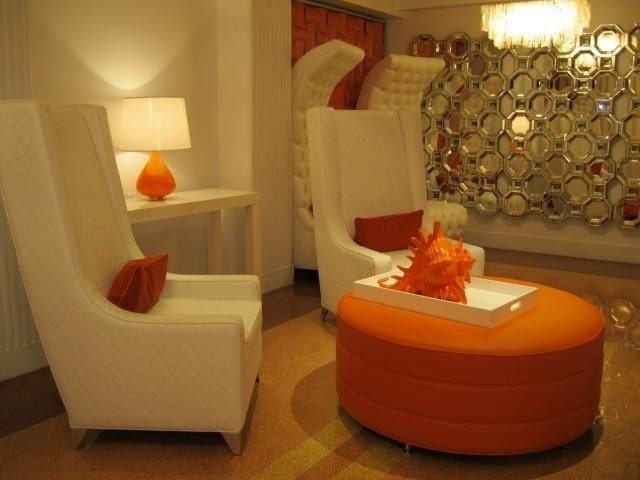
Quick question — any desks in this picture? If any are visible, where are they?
[123,183,261,280]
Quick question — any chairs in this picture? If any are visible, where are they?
[305,104,487,329]
[289,37,366,276]
[0,93,263,458]
[351,52,469,244]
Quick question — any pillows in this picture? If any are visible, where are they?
[351,207,427,254]
[106,251,169,318]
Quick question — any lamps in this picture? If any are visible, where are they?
[477,0,593,53]
[111,95,195,203]
